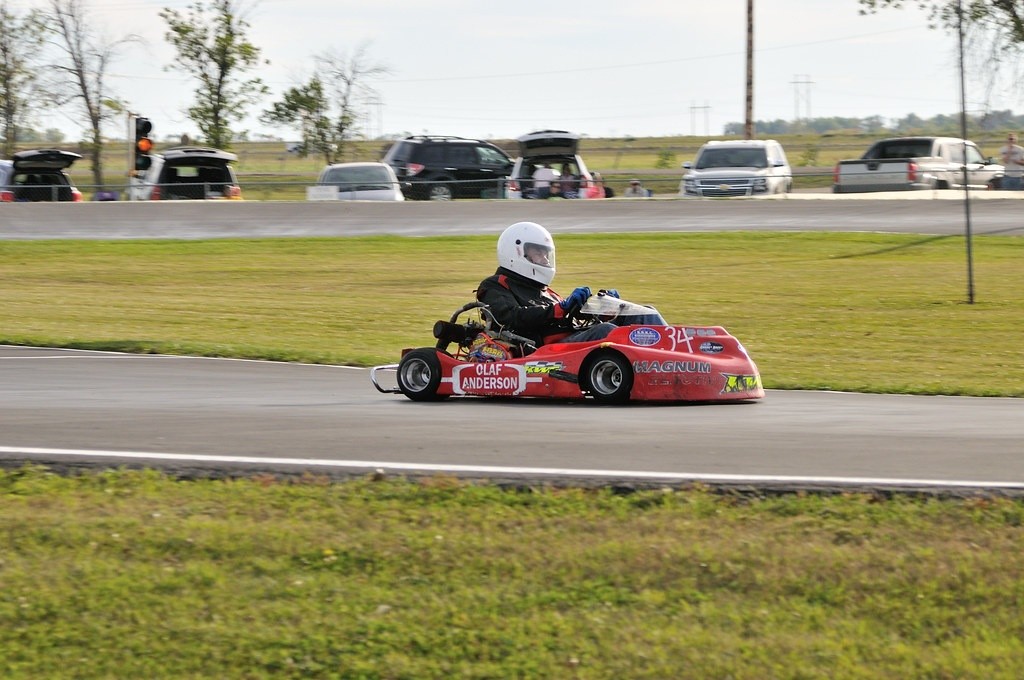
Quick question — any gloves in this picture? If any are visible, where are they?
[605,290,619,298]
[559,286,590,313]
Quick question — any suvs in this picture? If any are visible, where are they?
[381,135,554,201]
[679,139,793,197]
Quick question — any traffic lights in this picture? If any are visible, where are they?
[135,118,153,170]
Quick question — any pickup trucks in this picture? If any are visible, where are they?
[833,136,1004,194]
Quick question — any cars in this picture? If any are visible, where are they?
[505,129,595,199]
[317,162,405,203]
[0,149,84,202]
[126,146,240,200]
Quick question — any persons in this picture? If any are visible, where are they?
[477,222,666,342]
[624,178,648,197]
[679,162,702,194]
[533,161,612,198]
[1000,133,1024,190]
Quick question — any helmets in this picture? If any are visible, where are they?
[497,222,556,287]
